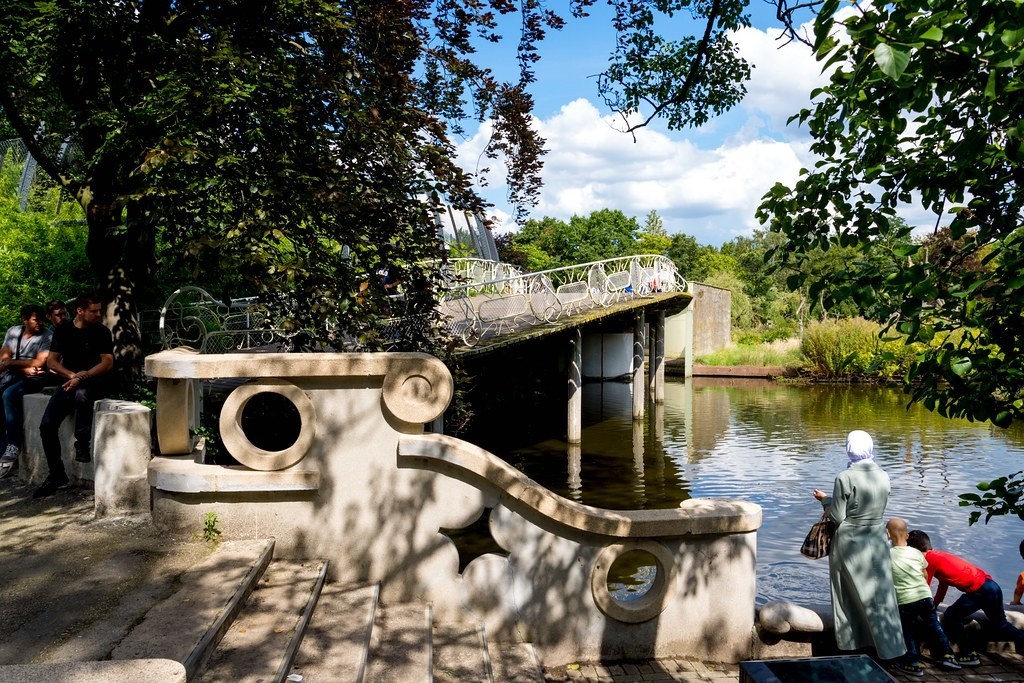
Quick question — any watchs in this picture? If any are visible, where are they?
[76,377,80,381]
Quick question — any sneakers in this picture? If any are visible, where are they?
[895,658,925,676]
[942,652,964,670]
[0,444,21,462]
[0,461,14,480]
[957,650,982,667]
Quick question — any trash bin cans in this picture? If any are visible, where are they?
[737,653,901,683]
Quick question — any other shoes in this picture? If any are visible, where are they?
[32,471,70,499]
[76,449,91,464]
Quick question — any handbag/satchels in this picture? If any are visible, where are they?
[799,506,839,561]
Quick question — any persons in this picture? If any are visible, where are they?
[0,291,114,497]
[382,268,401,294]
[882,517,1024,676]
[811,430,908,661]
